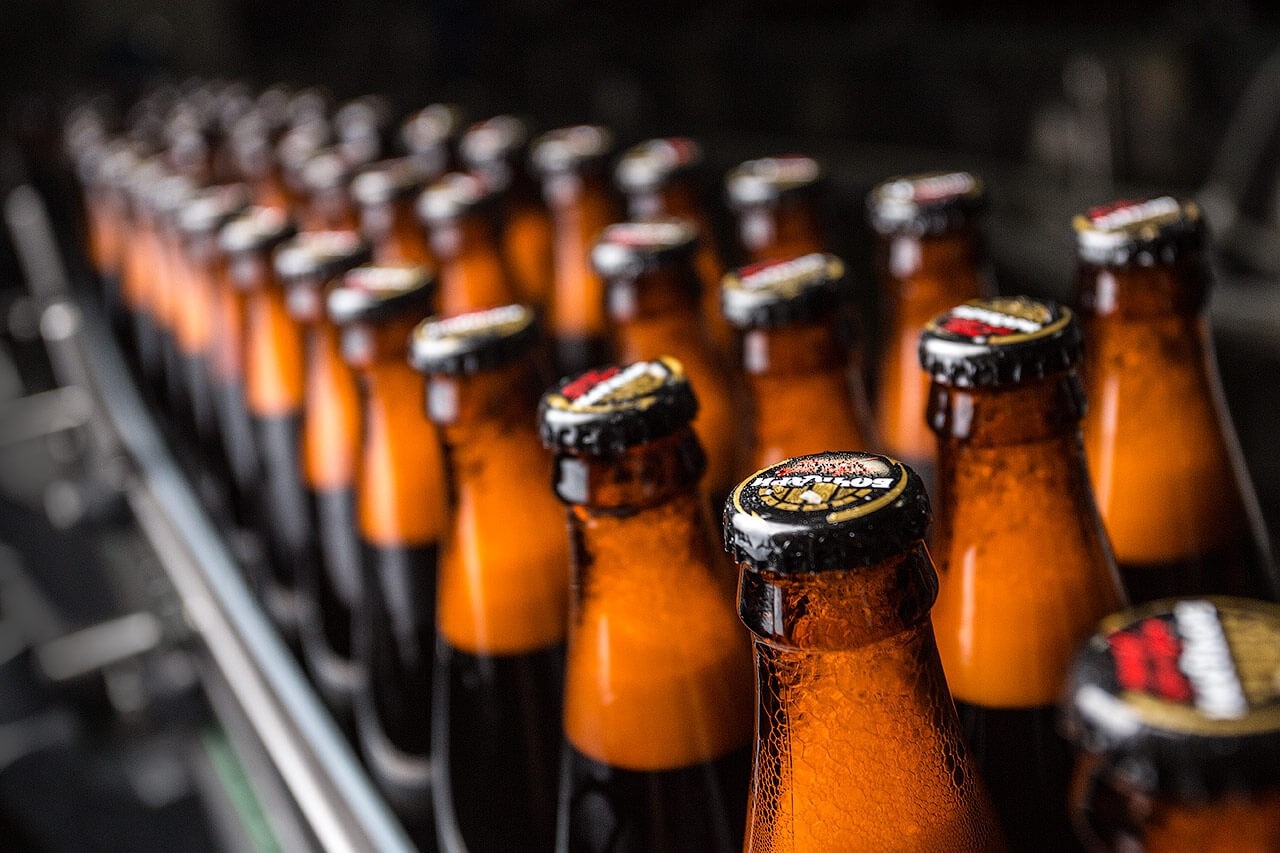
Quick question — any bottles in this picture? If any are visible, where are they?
[62,82,1277,853]
